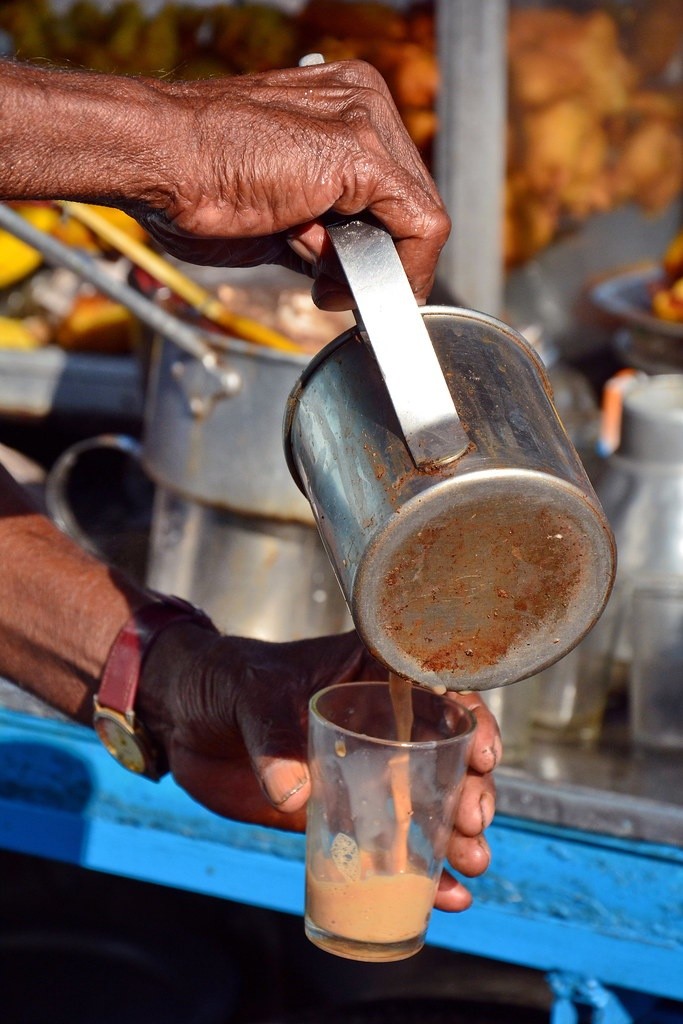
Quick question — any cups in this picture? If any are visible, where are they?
[46,280,363,646]
[306,682,476,963]
[283,52,618,695]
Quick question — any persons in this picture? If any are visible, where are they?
[0,61,505,911]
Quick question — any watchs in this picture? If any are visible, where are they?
[95,597,215,785]
